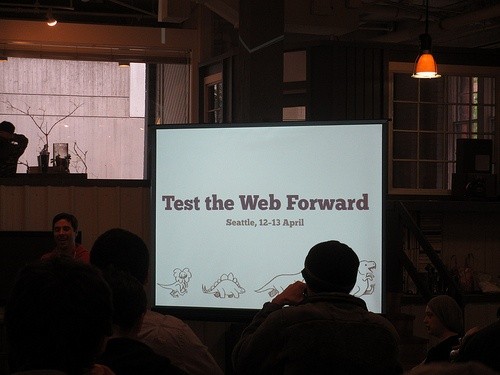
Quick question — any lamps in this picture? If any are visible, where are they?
[46,8,58,26]
[411,0,442,79]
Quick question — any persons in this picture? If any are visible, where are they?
[458,306,500,374]
[407,295,463,370]
[231,240,405,375]
[0,227,224,375]
[0,121,28,174]
[38,213,90,265]
